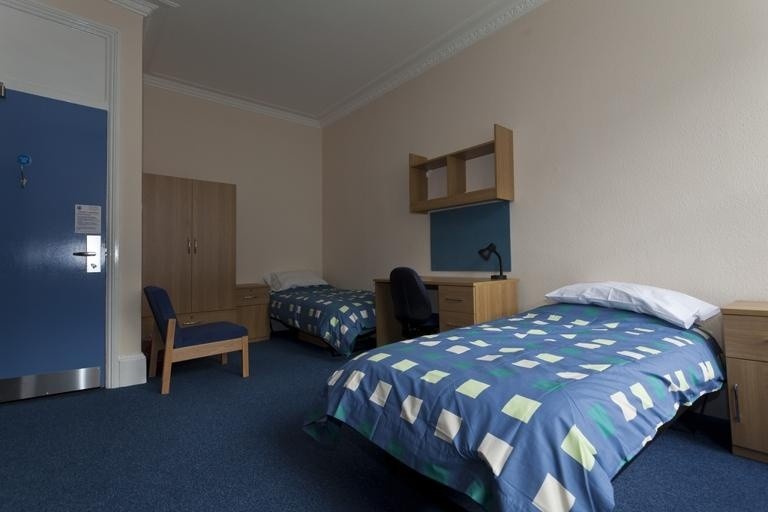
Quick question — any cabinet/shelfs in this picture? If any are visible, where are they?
[372,277,520,347]
[143,172,237,350]
[409,124,514,214]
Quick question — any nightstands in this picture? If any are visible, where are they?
[236,284,271,342]
[720,299,768,464]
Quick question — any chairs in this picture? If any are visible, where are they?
[144,286,250,394]
[390,267,439,338]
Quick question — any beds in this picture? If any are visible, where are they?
[269,285,377,359]
[301,304,727,512]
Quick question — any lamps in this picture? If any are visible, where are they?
[478,243,507,280]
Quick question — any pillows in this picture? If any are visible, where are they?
[545,281,720,328]
[263,271,329,293]
